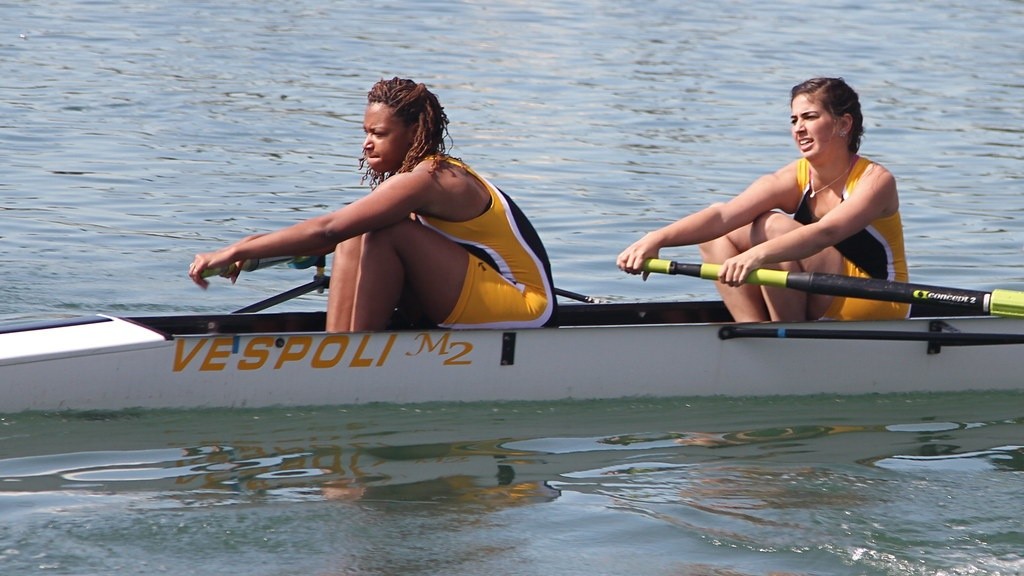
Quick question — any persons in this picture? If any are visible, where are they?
[188,76,558,332]
[616,77,910,323]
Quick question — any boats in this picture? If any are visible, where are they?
[0,305,1023,420]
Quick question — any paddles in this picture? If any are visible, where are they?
[187,245,336,281]
[639,257,1024,320]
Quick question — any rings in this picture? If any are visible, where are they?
[194,268,199,273]
